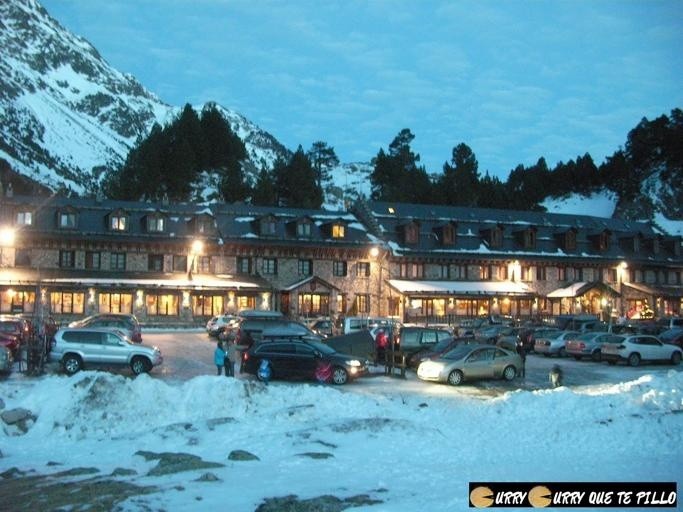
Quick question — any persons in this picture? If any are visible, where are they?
[527,332,532,353]
[225,338,235,378]
[222,323,232,339]
[214,341,225,375]
[285,306,299,322]
[516,340,526,376]
[520,332,527,346]
[328,309,338,326]
[615,311,626,324]
[375,328,388,363]
[548,363,563,388]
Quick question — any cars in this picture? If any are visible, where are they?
[407,337,525,387]
[1,313,58,375]
[457,316,682,367]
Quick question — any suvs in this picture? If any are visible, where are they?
[51,327,162,375]
[68,314,143,343]
[205,310,451,386]
